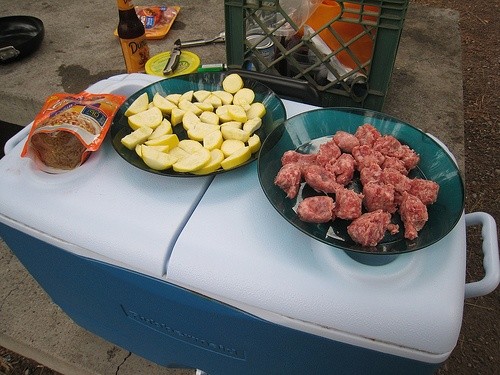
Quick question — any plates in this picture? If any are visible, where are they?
[257,108,465,255]
[111,73,286,177]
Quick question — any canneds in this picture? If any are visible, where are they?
[245,34,275,74]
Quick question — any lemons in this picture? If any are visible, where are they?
[120,74,266,175]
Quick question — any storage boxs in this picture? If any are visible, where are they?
[224,0,409,118]
[0,73,500,375]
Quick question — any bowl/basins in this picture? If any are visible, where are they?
[0,15,44,65]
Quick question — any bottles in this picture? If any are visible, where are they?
[117,0,150,74]
[316,54,331,87]
